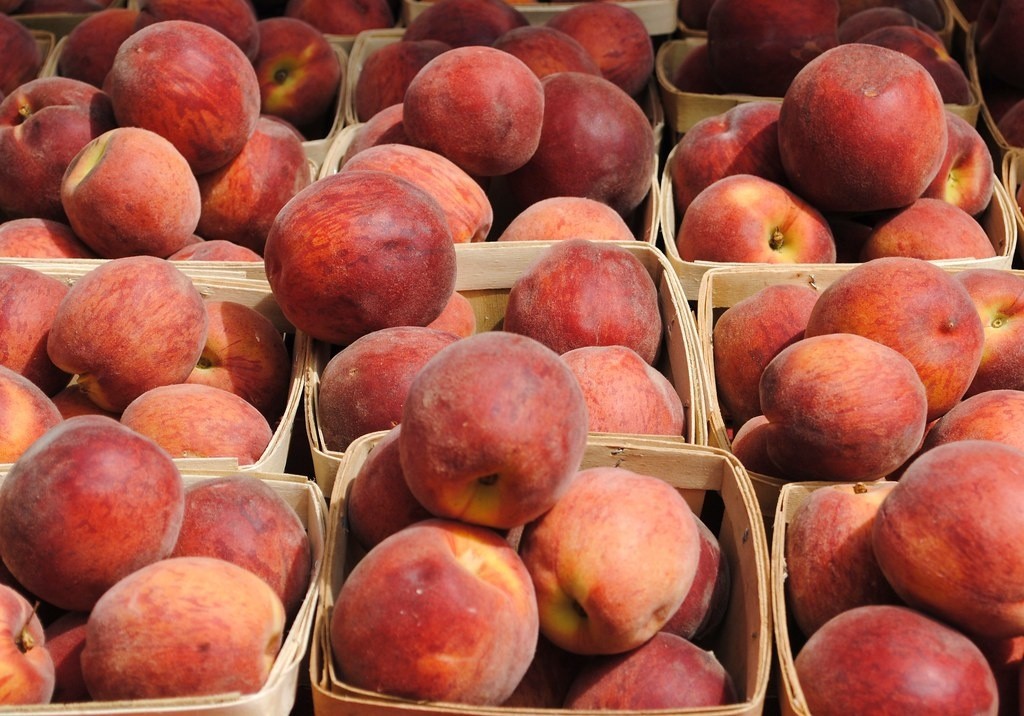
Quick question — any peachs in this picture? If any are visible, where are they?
[0,0,1024,716]
